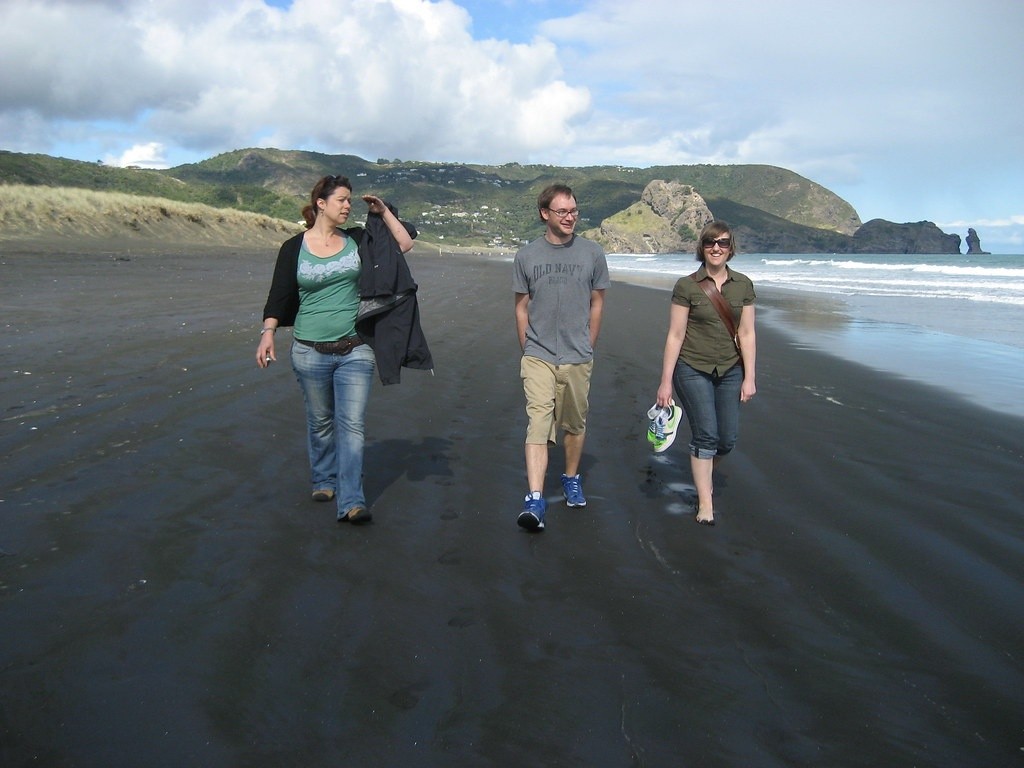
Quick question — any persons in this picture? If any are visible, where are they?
[655,219,757,524]
[511,183,611,530]
[256,175,414,524]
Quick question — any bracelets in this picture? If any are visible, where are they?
[260,327,276,335]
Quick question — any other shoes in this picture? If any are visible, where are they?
[311,489,334,502]
[347,505,373,525]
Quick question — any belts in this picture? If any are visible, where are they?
[294,336,364,356]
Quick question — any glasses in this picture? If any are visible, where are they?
[546,207,580,217]
[700,237,731,248]
[320,174,340,198]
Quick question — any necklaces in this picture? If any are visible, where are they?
[322,238,331,246]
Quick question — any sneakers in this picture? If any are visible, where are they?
[559,473,587,508]
[654,405,682,453]
[517,491,547,534]
[646,399,676,445]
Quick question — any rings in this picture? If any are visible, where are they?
[750,396,753,399]
